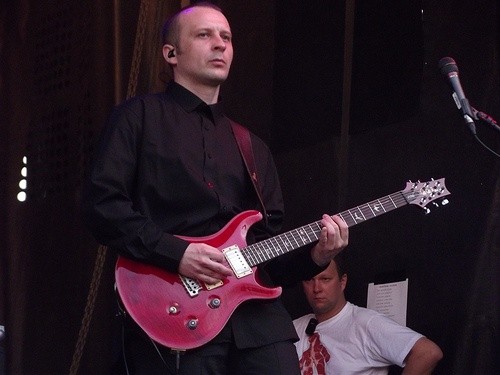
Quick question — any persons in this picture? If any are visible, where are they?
[84,2,349,375]
[292,252,443,375]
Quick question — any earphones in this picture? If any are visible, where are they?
[168,49,175,57]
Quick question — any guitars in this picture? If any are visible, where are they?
[115,177,451,349]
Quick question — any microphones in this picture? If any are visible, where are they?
[438,56,477,135]
